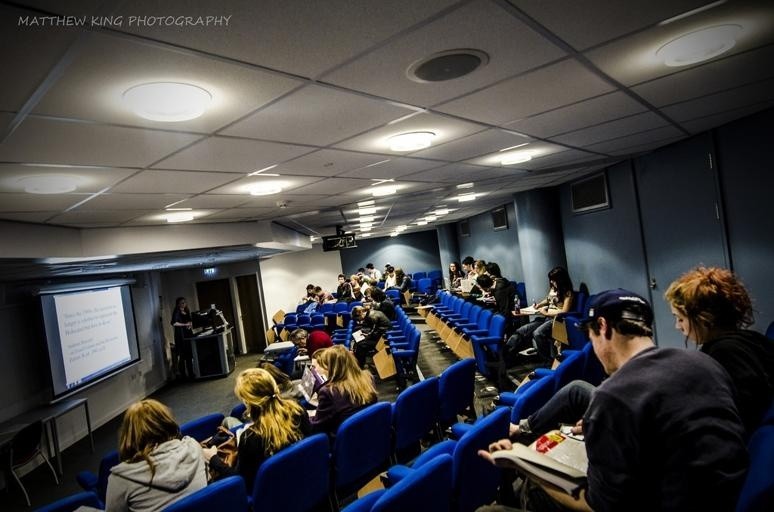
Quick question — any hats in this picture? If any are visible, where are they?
[575,287,656,324]
[305,330,334,360]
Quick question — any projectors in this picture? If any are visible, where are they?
[322,233,354,251]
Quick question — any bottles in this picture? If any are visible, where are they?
[514,295,521,315]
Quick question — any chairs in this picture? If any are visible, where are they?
[1,417,59,507]
[37,268,773,511]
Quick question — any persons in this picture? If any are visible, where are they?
[72,257,773,512]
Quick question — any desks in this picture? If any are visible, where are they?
[1,396,96,478]
